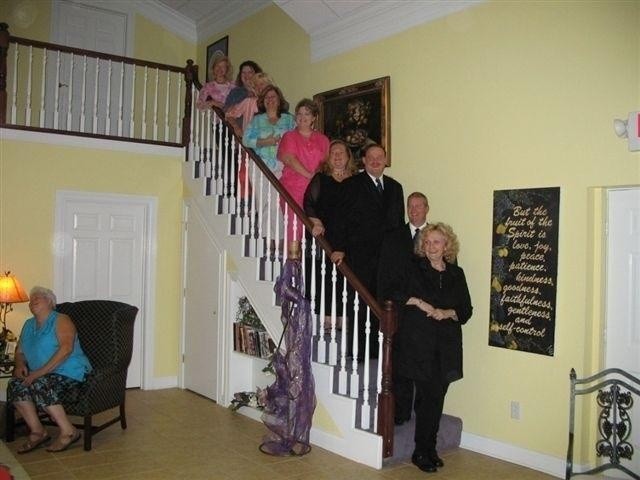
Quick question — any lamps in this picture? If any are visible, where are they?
[1,272,31,375]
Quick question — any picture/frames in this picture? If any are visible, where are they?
[207,36,228,82]
[312,77,390,169]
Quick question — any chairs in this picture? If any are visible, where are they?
[5,299,138,451]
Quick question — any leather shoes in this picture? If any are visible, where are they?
[428,444,444,467]
[411,448,437,473]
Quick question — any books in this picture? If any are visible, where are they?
[233,322,275,359]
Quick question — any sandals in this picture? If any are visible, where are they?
[18,429,48,453]
[47,425,82,451]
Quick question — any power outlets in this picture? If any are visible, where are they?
[510,401,522,423]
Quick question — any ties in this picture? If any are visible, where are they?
[413,229,420,241]
[376,176,383,196]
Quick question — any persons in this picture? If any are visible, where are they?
[196,56,235,183]
[330,144,405,363]
[381,193,457,426]
[276,98,329,262]
[303,140,359,336]
[6,286,93,453]
[224,61,269,198]
[385,222,473,474]
[242,84,297,257]
[225,72,277,217]
